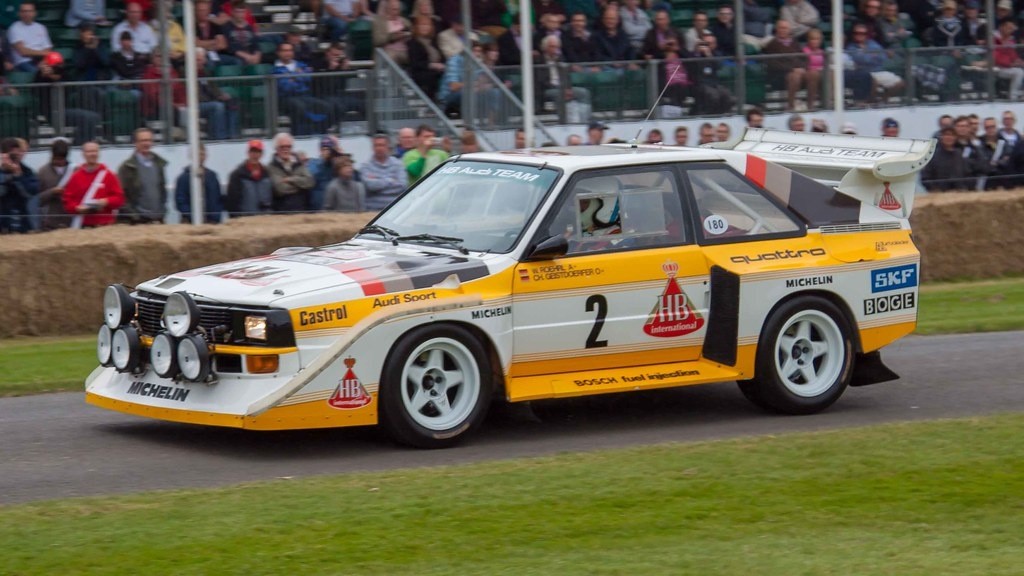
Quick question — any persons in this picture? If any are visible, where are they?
[360,133,409,211]
[61,140,126,229]
[1,137,79,233]
[267,133,316,213]
[227,139,275,218]
[119,127,168,224]
[552,175,637,254]
[307,135,366,211]
[0,1,1024,148]
[173,139,223,227]
[393,109,1024,192]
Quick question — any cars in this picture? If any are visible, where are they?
[81,129,943,445]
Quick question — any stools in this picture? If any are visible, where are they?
[0,0,1023,154]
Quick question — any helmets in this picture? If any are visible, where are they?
[574,176,623,227]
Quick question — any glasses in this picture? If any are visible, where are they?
[854,31,867,36]
[278,144,292,148]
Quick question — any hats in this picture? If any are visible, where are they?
[998,0,1012,10]
[965,0,980,9]
[942,0,957,9]
[45,52,62,66]
[589,120,610,130]
[248,140,264,153]
[842,122,856,134]
